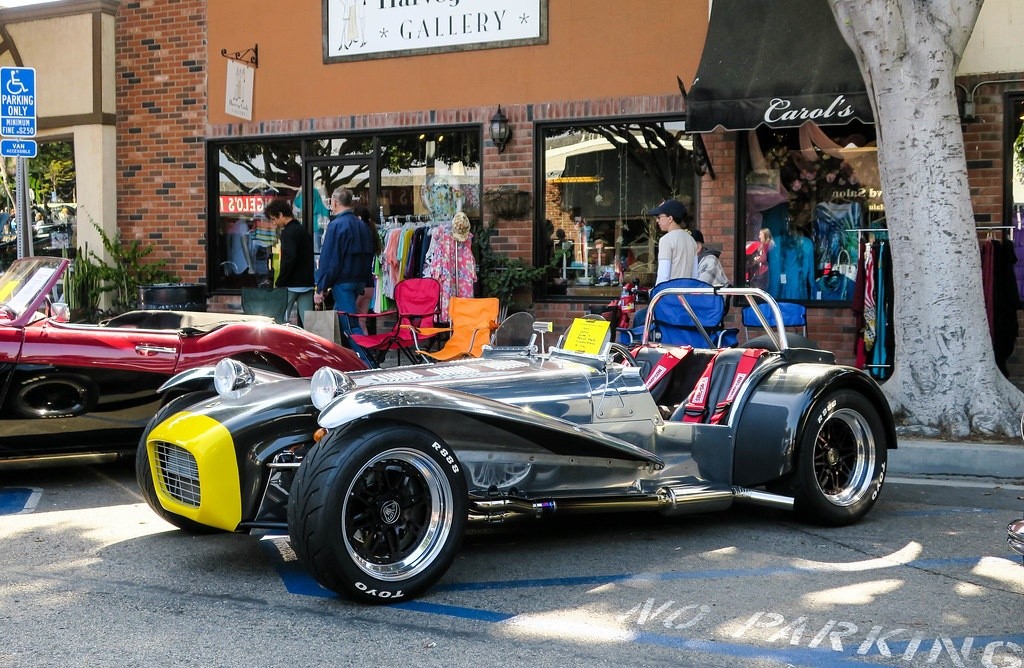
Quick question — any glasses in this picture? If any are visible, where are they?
[653,215,668,221]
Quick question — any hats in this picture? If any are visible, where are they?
[647,199,687,218]
[450,212,471,241]
[690,229,704,243]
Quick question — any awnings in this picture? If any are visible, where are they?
[686,0,875,134]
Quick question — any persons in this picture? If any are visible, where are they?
[314,186,381,369]
[749,228,776,290]
[264,199,314,327]
[647,200,699,294]
[35,213,44,234]
[424,165,462,215]
[354,207,377,336]
[230,213,256,293]
[688,230,729,306]
[546,220,560,282]
[555,229,575,280]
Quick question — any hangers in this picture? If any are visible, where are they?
[381,214,455,230]
[859,229,889,249]
[985,227,1011,242]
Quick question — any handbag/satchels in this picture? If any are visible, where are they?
[364,272,375,287]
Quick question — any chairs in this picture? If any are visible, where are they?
[670,347,770,425]
[625,345,674,400]
[337,277,808,364]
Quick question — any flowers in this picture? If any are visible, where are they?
[764,133,881,193]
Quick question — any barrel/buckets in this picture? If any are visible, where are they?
[135,282,207,312]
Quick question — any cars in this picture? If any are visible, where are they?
[0,256,371,462]
[134,288,898,606]
[34,224,68,239]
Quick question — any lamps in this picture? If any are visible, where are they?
[488,104,512,155]
